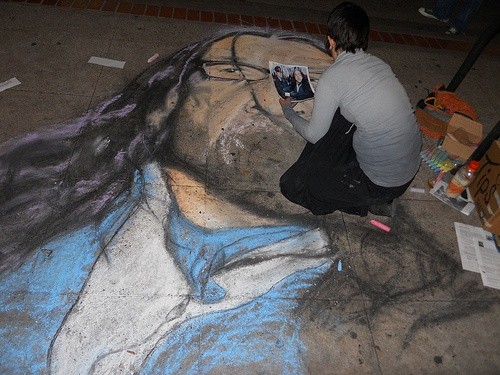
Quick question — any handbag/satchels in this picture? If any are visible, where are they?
[415,83,477,139]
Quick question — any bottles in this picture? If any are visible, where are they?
[446,160,480,197]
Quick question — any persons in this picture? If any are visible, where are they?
[274,66,314,99]
[279,2,422,216]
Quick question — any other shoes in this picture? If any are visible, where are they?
[369,196,398,218]
